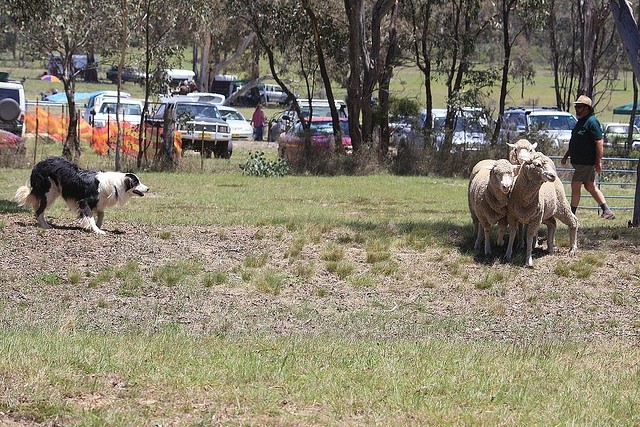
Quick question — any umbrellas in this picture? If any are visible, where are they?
[40,74,62,86]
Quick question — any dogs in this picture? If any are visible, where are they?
[14,156,150,235]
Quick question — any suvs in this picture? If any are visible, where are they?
[284,96,348,131]
[0,81,27,137]
[258,84,296,105]
[150,101,232,159]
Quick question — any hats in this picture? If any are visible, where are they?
[571,95,594,112]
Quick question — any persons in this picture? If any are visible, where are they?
[560,95,615,220]
[249,103,266,142]
[50,88,58,95]
[43,94,50,101]
[169,78,198,98]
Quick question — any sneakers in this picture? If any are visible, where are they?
[601,209,615,220]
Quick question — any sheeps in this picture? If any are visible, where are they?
[505,139,540,250]
[504,152,579,267]
[468,159,521,255]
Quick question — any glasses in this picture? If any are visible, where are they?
[574,105,585,109]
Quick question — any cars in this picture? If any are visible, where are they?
[106,65,153,84]
[267,109,294,141]
[93,99,145,127]
[601,123,640,149]
[214,106,253,141]
[278,117,363,162]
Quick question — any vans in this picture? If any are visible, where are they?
[82,89,130,128]
[393,109,496,164]
[496,107,579,152]
